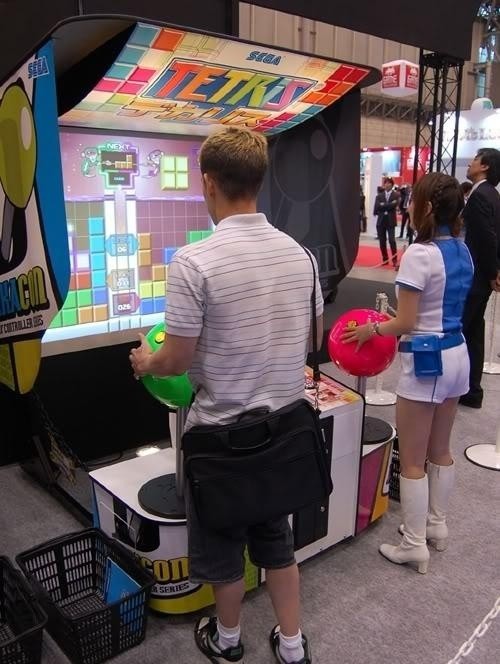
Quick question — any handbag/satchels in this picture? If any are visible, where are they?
[180,399,333,544]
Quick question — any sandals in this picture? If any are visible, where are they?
[194,616,245,664]
[270,623,311,664]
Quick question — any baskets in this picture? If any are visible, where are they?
[0,555,49,664]
[15,526,156,664]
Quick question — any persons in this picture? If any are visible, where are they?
[454,181,474,242]
[336,171,475,572]
[356,179,414,266]
[455,146,500,408]
[130,125,326,664]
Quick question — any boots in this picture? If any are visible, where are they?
[399,458,456,551]
[379,472,430,574]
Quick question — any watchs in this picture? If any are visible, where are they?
[371,320,386,337]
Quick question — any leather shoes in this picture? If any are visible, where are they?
[460,395,481,409]
